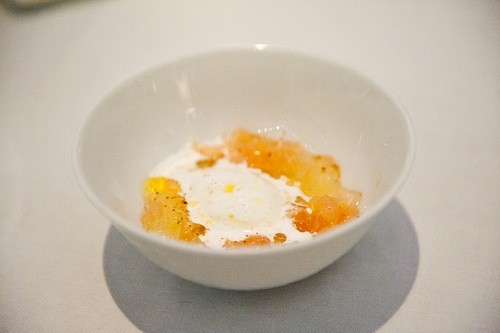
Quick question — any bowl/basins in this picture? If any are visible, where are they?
[75,44,415,292]
